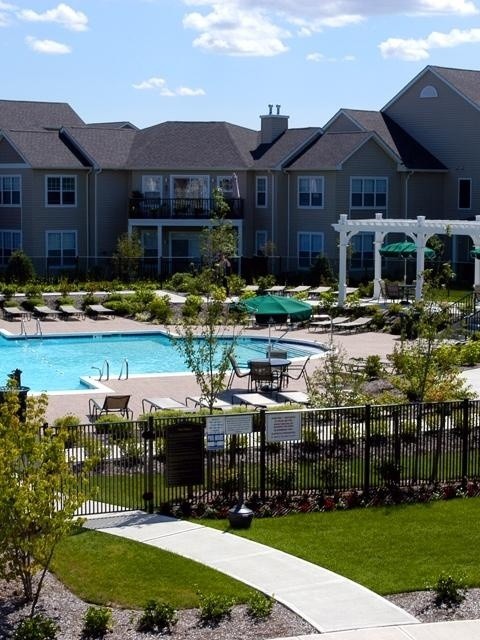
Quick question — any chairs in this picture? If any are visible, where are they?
[240,284,361,298]
[308,316,374,334]
[331,359,396,372]
[4,304,116,322]
[88,390,314,421]
[377,279,416,305]
[225,348,312,393]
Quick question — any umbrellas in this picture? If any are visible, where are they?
[471,248,480,260]
[379,240,437,284]
[229,294,314,358]
